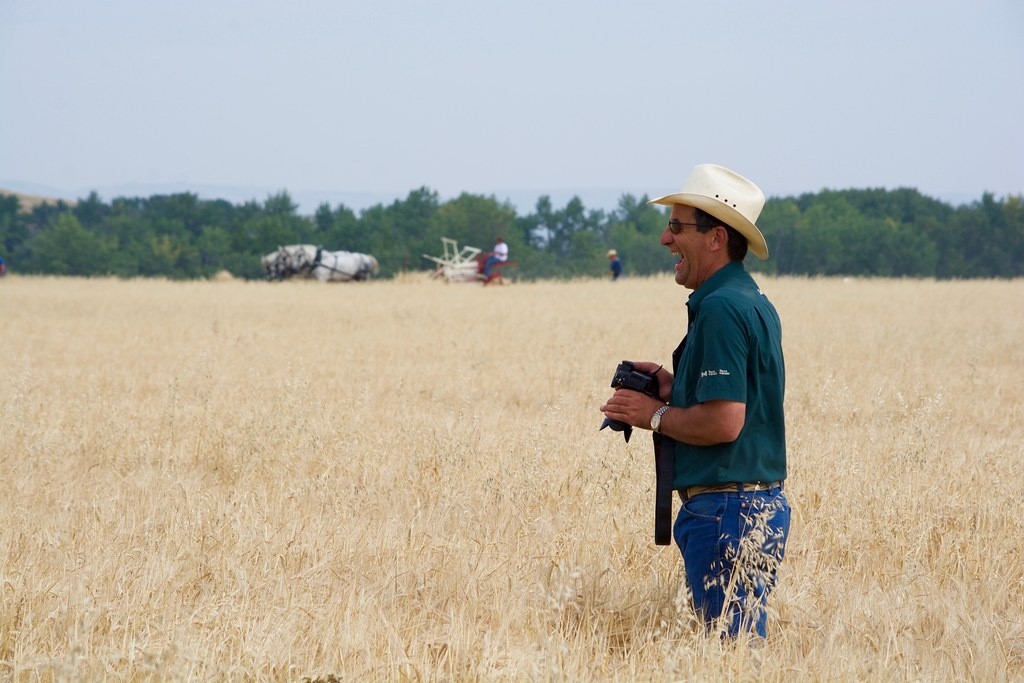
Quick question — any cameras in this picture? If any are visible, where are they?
[599,364,657,443]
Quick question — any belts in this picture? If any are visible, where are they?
[678,480,784,500]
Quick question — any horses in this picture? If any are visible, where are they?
[261,244,379,282]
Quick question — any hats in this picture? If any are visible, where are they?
[606,250,617,257]
[646,164,768,260]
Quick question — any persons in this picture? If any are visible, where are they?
[601,163,791,643]
[483,236,508,276]
[606,248,623,281]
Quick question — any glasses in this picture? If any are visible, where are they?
[669,218,712,234]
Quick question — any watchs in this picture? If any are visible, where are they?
[650,405,670,432]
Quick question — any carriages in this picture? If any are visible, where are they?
[260,239,520,287]
